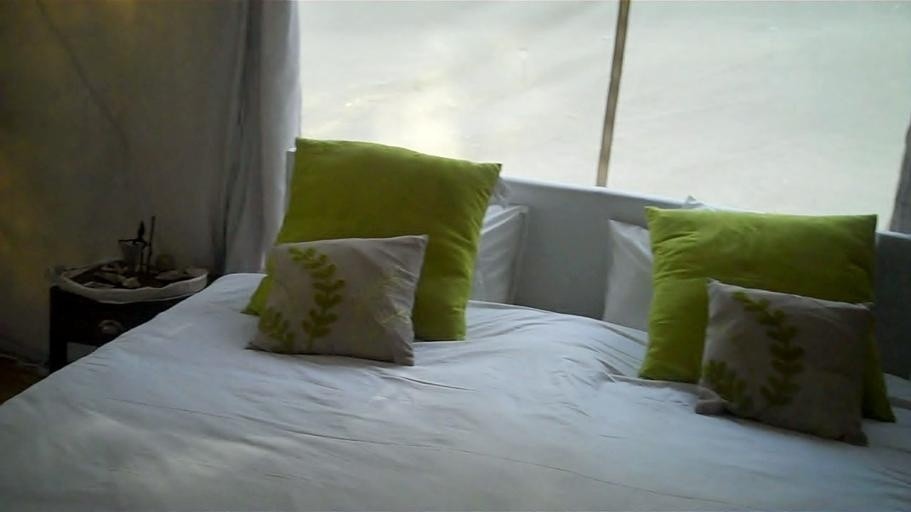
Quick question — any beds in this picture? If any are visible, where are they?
[1,145,910,512]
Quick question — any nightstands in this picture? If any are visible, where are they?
[49,264,217,373]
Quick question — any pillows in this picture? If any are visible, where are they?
[241,137,502,340]
[472,202,526,304]
[603,215,655,332]
[693,277,876,444]
[245,235,430,366]
[636,207,897,424]
